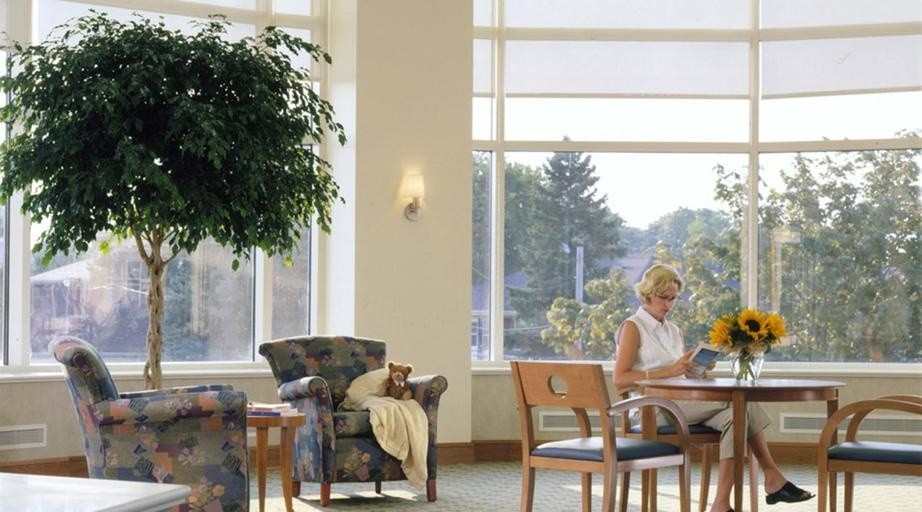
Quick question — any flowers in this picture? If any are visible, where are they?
[707,305,789,381]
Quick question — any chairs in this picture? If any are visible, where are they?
[815,392,922,512]
[47,333,250,512]
[615,373,767,512]
[507,357,695,512]
[253,328,450,509]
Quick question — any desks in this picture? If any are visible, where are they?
[244,408,308,512]
[0,469,192,512]
[633,374,848,512]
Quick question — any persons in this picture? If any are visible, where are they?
[612,263,816,511]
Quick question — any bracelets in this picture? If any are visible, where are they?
[645,371,649,379]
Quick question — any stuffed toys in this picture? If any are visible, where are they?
[377,361,413,400]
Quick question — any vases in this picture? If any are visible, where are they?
[725,348,766,386]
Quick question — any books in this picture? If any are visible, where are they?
[684,342,720,380]
[248,401,292,411]
[247,407,299,416]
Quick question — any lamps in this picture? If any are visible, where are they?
[397,171,428,223]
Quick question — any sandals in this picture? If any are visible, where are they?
[766,480,817,505]
